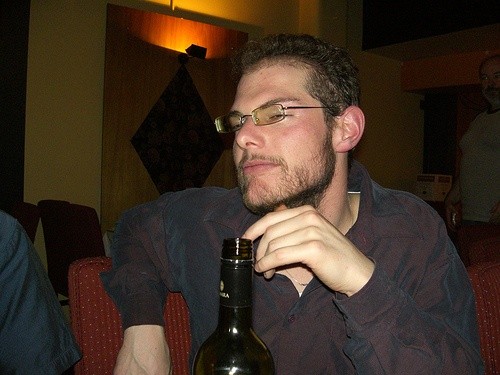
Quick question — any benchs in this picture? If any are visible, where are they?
[69,256,500,375]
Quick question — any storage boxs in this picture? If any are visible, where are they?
[415,173,452,201]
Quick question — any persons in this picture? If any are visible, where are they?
[0,209,85,375]
[99,33,485,375]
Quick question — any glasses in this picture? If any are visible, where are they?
[215,104,347,133]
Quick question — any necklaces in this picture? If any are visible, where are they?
[284,265,308,286]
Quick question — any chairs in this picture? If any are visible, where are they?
[38,200,106,306]
[1,201,41,243]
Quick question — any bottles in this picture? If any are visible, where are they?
[192,237,276,375]
